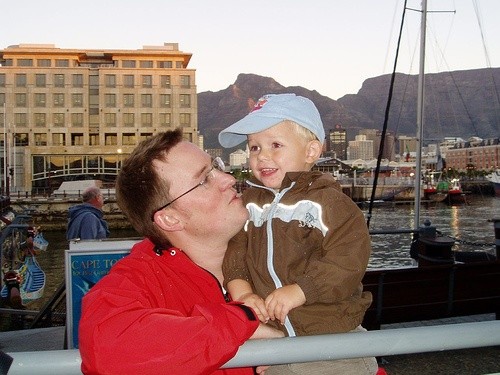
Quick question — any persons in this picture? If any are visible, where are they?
[64,94,387,375]
[222,94,378,375]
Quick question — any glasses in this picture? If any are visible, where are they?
[151,156,226,222]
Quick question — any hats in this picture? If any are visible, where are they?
[218,93,325,148]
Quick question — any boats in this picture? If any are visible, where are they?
[423,167,463,204]
[0,226,49,308]
[484,168,500,196]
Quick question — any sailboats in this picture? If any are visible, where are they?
[359,1,500,323]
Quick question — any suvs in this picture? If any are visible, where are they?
[51,180,103,200]
